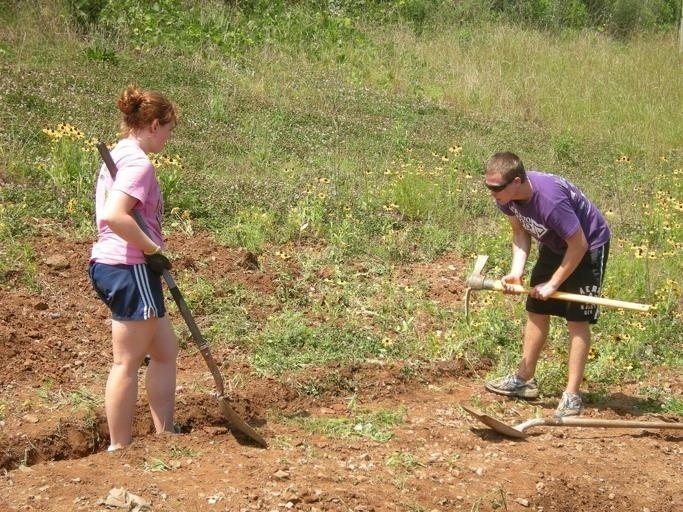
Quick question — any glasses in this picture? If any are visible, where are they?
[484,178,514,192]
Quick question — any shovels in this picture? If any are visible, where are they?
[461,403,683,439]
[95,139,268,450]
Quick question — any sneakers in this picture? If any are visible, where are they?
[485,372,540,398]
[555,391,582,417]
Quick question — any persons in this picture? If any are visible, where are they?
[484,151,612,417]
[88,82,181,453]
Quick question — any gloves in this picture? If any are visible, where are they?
[144,246,172,275]
